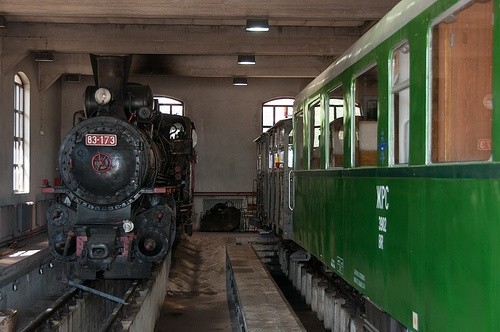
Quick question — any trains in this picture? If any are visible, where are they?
[252,0,500,332]
[37,54,198,282]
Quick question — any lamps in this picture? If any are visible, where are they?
[238,55,256,65]
[63,75,80,82]
[232,78,247,86]
[33,53,54,61]
[0,17,8,28]
[245,19,269,32]
